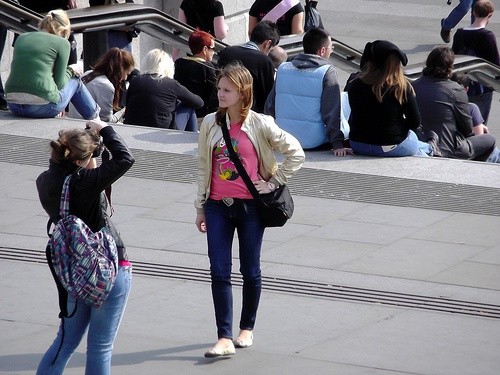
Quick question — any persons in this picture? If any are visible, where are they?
[0,0,500,164]
[193,64,304,358]
[34,120,135,375]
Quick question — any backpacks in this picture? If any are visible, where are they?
[42,174,119,318]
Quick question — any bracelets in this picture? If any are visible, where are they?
[67,66,74,76]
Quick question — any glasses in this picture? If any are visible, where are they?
[208,46,217,52]
[322,46,335,51]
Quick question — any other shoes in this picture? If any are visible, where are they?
[204,346,237,358]
[441,19,451,43]
[234,329,253,347]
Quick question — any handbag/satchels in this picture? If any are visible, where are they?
[256,185,294,227]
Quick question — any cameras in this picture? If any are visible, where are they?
[84,127,103,157]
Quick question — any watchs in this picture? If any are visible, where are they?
[267,180,277,192]
